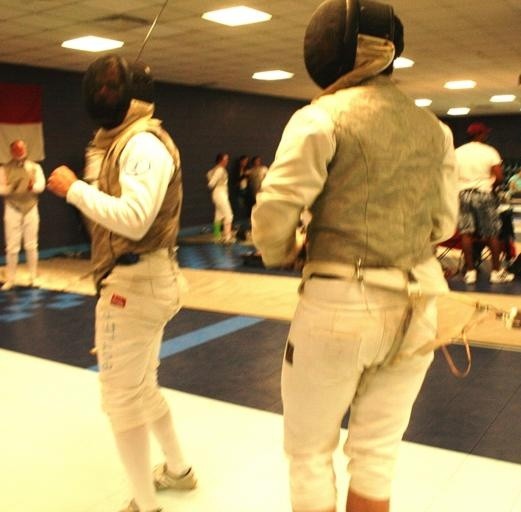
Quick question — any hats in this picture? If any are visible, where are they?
[467,123,493,137]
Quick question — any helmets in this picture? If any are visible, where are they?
[304,0,403,94]
[11,140,27,161]
[81,54,154,128]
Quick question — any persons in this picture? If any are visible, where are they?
[206,154,234,240]
[454,124,516,284]
[227,156,250,240]
[45,53,196,512]
[0,139,47,290]
[244,156,268,200]
[250,0,460,510]
[505,168,520,196]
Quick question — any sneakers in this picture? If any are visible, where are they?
[30,279,40,288]
[152,463,197,490]
[463,270,478,283]
[121,499,163,512]
[1,281,16,291]
[490,270,515,284]
